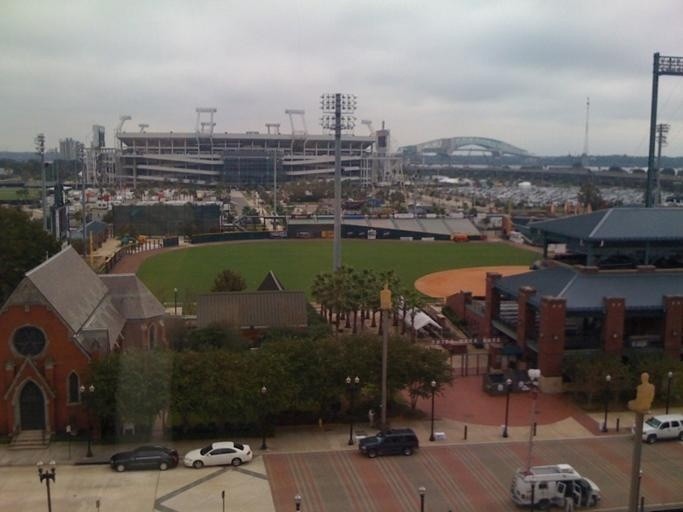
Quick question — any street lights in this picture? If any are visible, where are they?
[261,386,267,450]
[295,494,302,512]
[36,460,55,512]
[322,94,357,272]
[497,379,524,437]
[80,384,95,457]
[430,380,436,440]
[35,134,47,236]
[666,372,672,414]
[646,52,681,207]
[173,287,178,315]
[346,376,359,445]
[602,375,612,432]
[418,487,427,511]
[519,368,542,470]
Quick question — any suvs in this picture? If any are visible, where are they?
[359,429,419,458]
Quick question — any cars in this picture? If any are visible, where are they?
[632,414,683,444]
[111,445,179,472]
[183,441,253,468]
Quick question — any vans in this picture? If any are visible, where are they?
[510,465,601,510]
